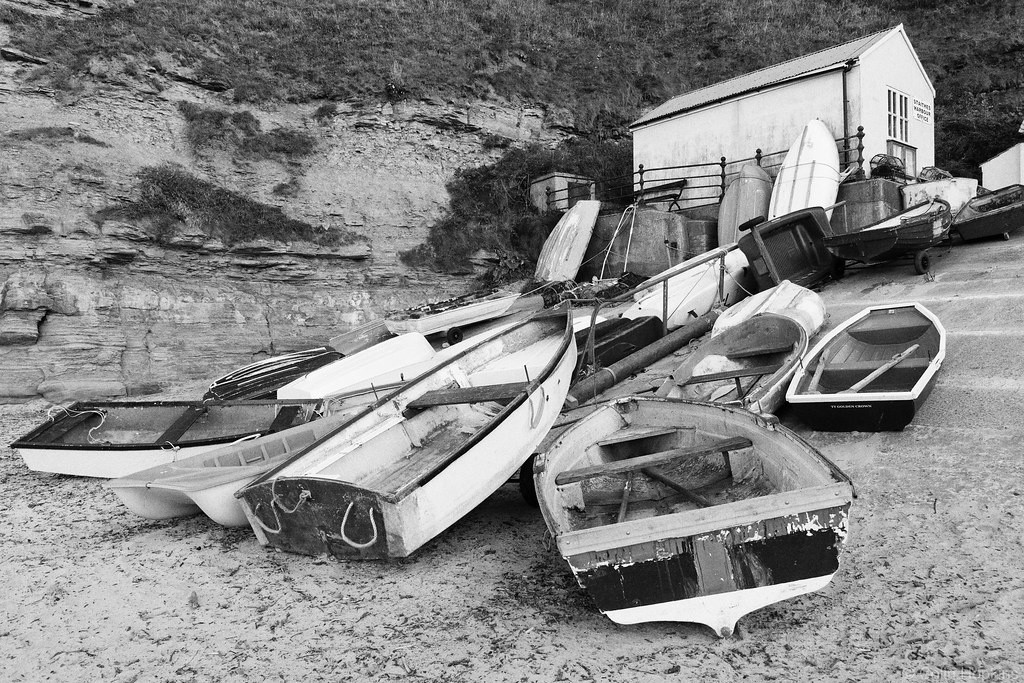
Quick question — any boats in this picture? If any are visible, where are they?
[99,397,376,530]
[10,394,384,480]
[529,393,860,642]
[822,194,954,275]
[534,200,601,285]
[951,183,1024,242]
[734,204,847,297]
[710,278,832,354]
[206,241,755,436]
[767,118,842,232]
[785,302,946,433]
[655,312,809,420]
[717,162,774,254]
[253,301,579,561]
